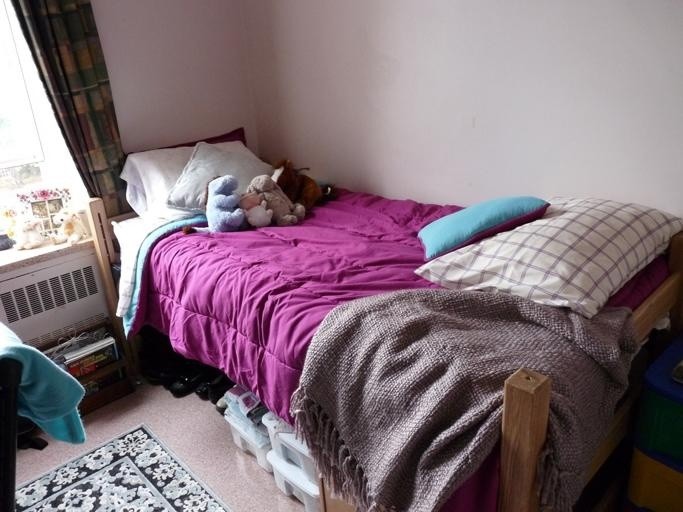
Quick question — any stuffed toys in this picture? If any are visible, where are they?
[13,216,42,250]
[238,190,274,227]
[199,175,248,233]
[247,174,305,226]
[51,207,86,244]
[271,158,336,210]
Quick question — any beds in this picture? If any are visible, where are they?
[82,199,683,511]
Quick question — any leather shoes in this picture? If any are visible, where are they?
[147,353,235,409]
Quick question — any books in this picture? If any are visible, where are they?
[63,336,125,398]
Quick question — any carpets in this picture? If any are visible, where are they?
[15,422,232,512]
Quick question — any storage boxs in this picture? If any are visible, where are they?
[218,402,323,511]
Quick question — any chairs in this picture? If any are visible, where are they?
[0,360,24,511]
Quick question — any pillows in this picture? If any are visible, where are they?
[413,195,683,319]
[120,139,275,222]
